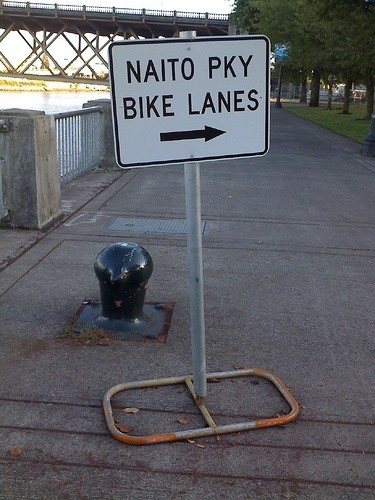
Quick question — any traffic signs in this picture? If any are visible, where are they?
[107,35,270,170]
